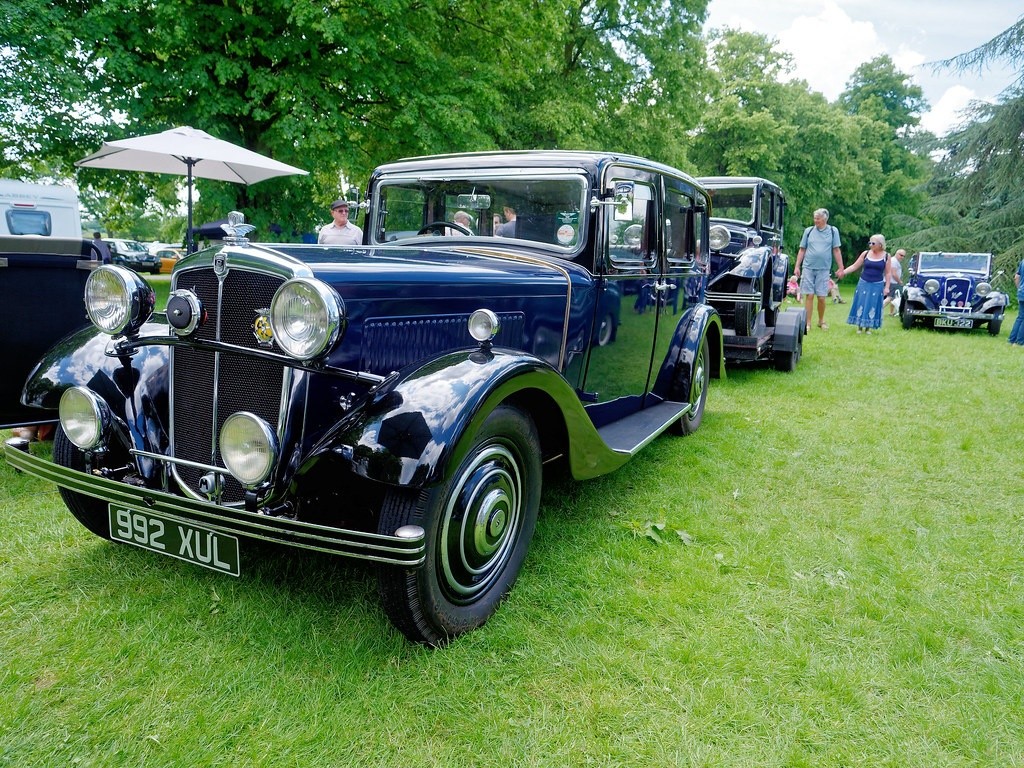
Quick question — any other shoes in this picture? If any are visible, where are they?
[838,299,846,304]
[888,313,896,316]
[856,329,871,334]
[832,299,837,304]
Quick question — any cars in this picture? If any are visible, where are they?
[100,238,162,276]
[154,248,189,276]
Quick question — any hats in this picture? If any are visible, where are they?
[331,200,348,210]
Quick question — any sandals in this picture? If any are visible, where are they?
[817,321,829,330]
[807,324,811,331]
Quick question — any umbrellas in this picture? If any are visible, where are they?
[73,125,309,258]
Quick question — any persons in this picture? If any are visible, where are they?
[788,275,801,302]
[317,201,364,245]
[89,231,112,264]
[445,205,518,238]
[1010,258,1024,347]
[794,208,906,336]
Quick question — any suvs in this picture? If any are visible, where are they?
[898,250,1009,338]
[4,145,729,652]
[620,175,808,372]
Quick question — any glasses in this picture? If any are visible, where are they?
[900,254,905,257]
[869,242,876,246]
[334,209,349,213]
